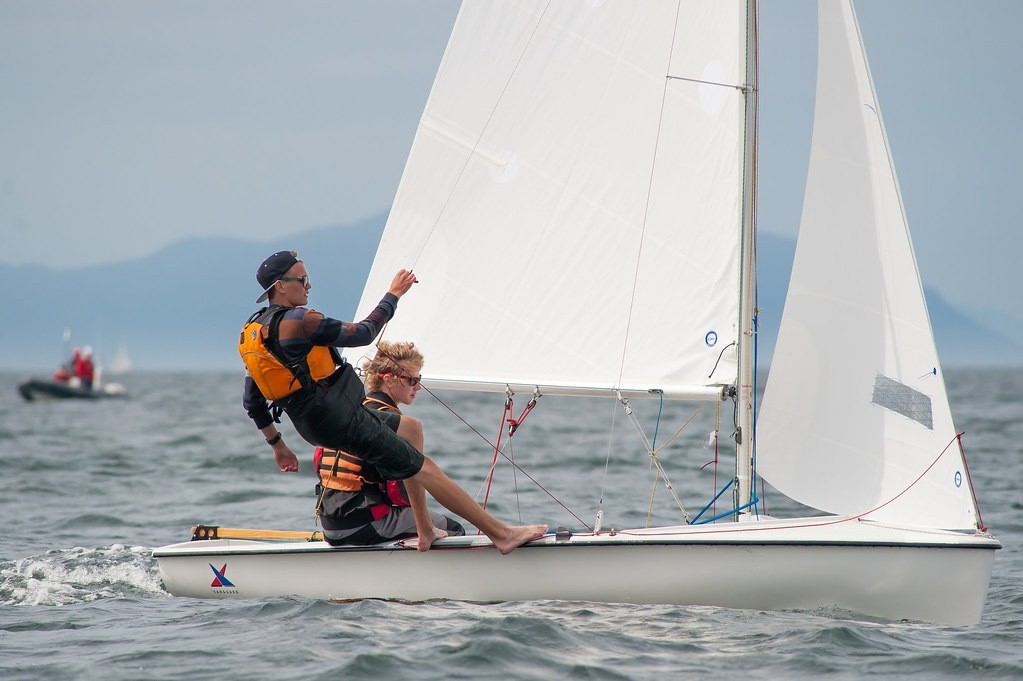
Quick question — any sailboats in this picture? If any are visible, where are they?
[141,0,998,634]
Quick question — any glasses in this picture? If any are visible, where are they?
[280,276,309,287]
[377,372,422,386]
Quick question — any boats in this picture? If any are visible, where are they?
[17,378,130,405]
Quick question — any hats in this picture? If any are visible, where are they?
[256,251,299,303]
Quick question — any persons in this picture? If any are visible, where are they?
[242,252,549,555]
[53,347,93,390]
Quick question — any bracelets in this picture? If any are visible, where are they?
[265,432,281,445]
